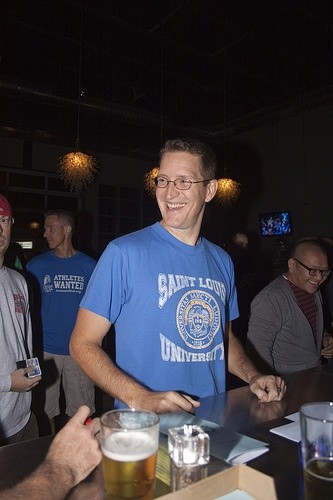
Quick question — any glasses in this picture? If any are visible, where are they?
[289,257,330,277]
[153,176,212,190]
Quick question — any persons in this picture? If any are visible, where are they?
[0,406,105,499]
[0,194,42,446]
[24,208,98,438]
[246,240,333,378]
[68,136,287,435]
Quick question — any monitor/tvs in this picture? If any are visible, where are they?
[259,211,293,237]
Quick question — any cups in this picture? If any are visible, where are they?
[99,408,160,500]
[299,401,333,500]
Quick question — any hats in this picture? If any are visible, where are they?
[0,194,12,217]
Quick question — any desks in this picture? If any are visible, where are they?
[0,362,333,500]
